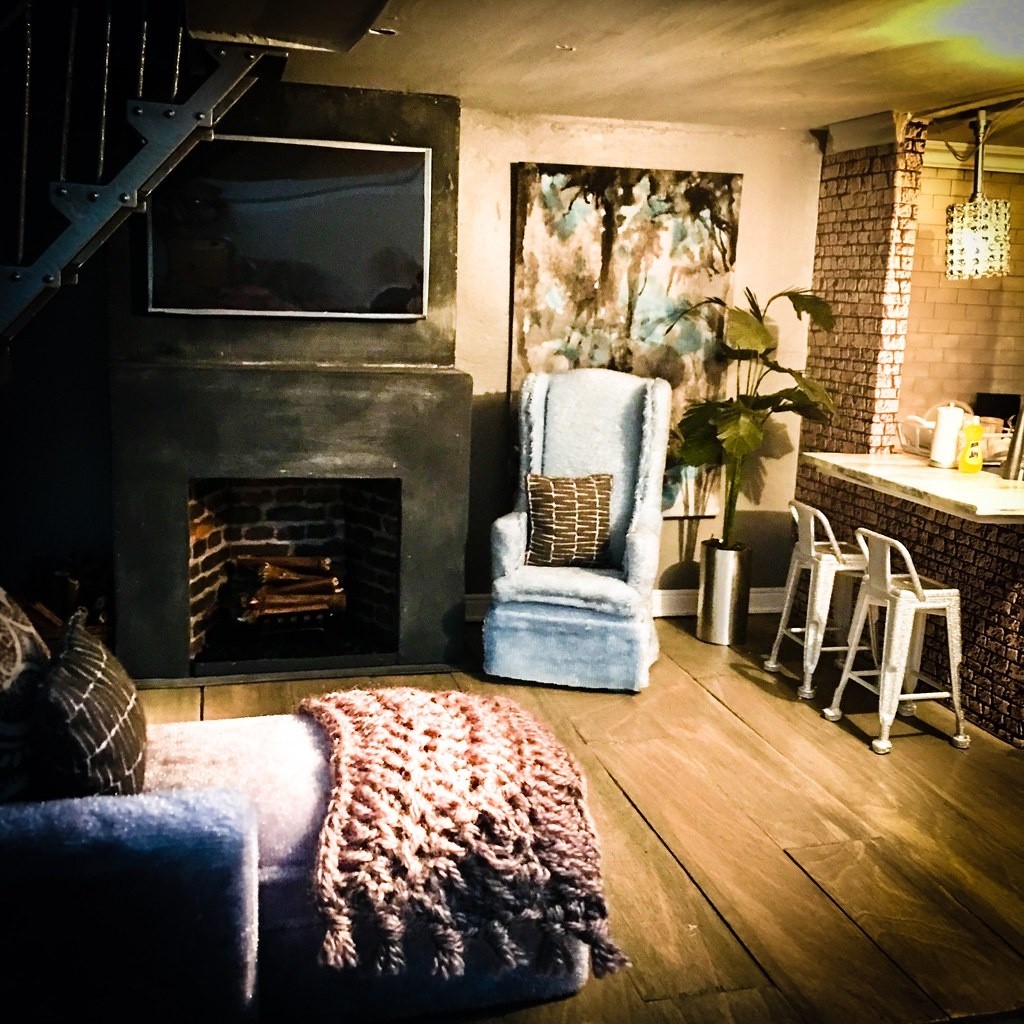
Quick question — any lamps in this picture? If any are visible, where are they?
[940,110,1013,282]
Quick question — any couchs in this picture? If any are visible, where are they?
[479,366,675,695]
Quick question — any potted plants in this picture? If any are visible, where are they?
[662,283,846,647]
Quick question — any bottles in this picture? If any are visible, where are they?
[929,402,966,464]
[958,416,984,473]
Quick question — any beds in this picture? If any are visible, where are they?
[6,683,627,999]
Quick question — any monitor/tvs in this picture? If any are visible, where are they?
[146,135,431,319]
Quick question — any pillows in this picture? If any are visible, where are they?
[31,610,149,800]
[523,472,614,568]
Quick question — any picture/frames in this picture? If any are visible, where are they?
[507,160,744,519]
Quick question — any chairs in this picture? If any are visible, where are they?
[764,499,882,698]
[827,528,974,756]
